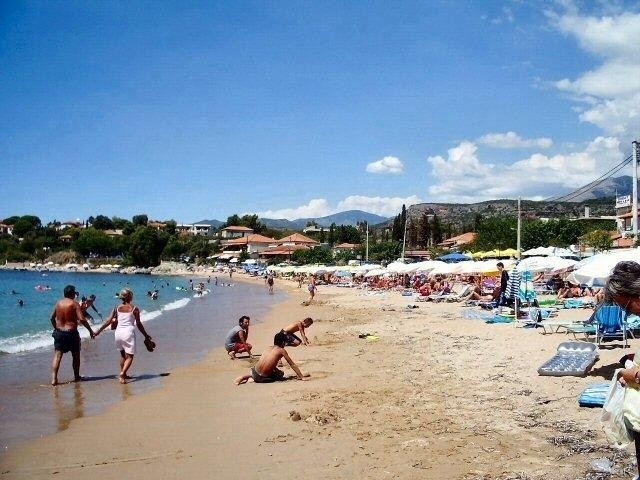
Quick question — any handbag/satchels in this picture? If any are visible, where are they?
[111,317,118,330]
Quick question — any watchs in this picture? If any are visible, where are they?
[634,370,638,385]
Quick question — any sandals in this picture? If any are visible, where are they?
[144,335,155,352]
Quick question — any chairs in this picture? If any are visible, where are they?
[421,281,640,350]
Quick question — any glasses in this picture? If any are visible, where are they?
[619,296,633,315]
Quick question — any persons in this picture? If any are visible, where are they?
[279,318,314,347]
[92,287,152,385]
[9,261,605,324]
[49,286,96,387]
[605,261,639,480]
[233,332,312,385]
[223,315,255,360]
